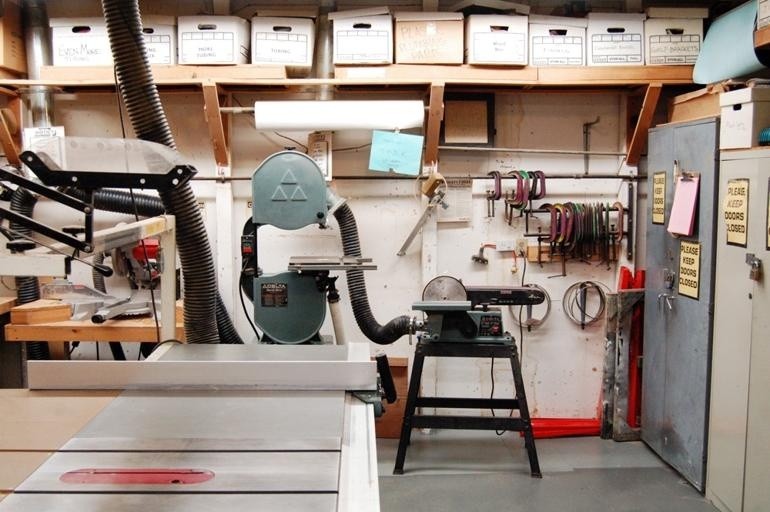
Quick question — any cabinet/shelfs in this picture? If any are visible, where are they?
[705,146,769,511]
[639,115,718,497]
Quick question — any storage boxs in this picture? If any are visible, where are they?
[448,0,530,67]
[393,9,464,66]
[528,12,588,66]
[586,11,646,67]
[719,88,770,150]
[178,14,250,66]
[644,5,709,65]
[48,15,116,67]
[327,5,394,66]
[140,13,178,65]
[0,0,28,77]
[250,8,318,77]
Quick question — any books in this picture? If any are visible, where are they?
[661,174,703,238]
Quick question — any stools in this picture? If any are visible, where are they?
[394,332,542,478]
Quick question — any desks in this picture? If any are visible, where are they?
[4,298,185,360]
